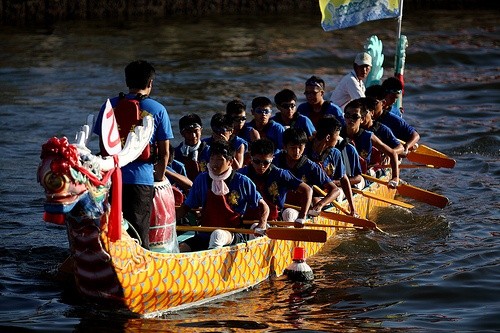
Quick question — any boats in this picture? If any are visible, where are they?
[36,31,409,320]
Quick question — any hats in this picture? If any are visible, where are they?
[354,52,373,66]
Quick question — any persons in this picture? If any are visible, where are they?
[92,59,174,250]
[328,52,373,114]
[157,75,420,253]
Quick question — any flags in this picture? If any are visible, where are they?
[318,0,403,32]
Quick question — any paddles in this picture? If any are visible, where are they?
[346,189,414,209]
[359,171,448,210]
[417,144,448,158]
[312,183,352,217]
[174,223,328,243]
[404,150,460,171]
[364,163,415,170]
[279,200,378,229]
[243,221,363,230]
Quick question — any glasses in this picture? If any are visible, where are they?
[256,108,272,113]
[280,103,296,109]
[361,112,367,118]
[336,123,342,131]
[216,145,228,155]
[233,115,247,122]
[344,113,361,120]
[306,80,321,87]
[186,124,195,129]
[216,127,226,134]
[390,89,402,94]
[375,100,379,105]
[253,158,271,165]
